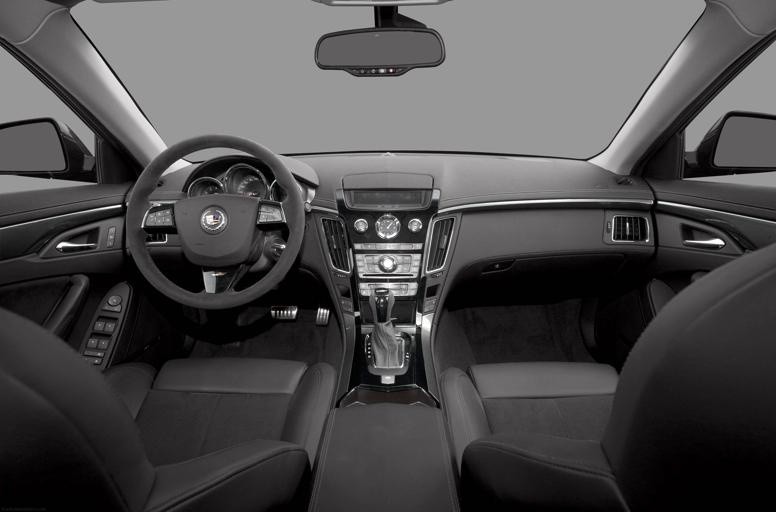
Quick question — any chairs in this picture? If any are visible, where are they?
[0,308,336,511]
[440,242,774,511]
